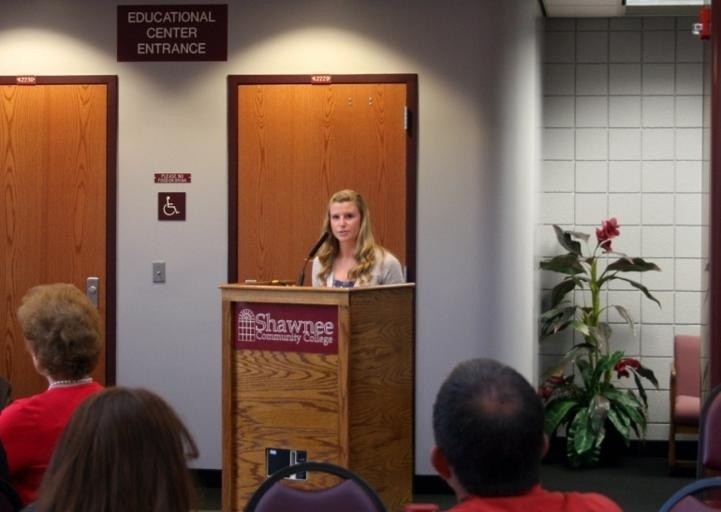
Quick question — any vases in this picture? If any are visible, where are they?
[561,406,628,468]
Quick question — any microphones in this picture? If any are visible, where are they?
[298,232,329,286]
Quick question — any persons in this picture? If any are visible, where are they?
[430,358,624,512]
[0,283,106,512]
[312,189,406,288]
[36,386,199,512]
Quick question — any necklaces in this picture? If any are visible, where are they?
[45,374,93,387]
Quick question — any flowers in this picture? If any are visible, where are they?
[540,217,661,470]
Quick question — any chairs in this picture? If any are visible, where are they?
[241,460,388,512]
[668,335,703,471]
